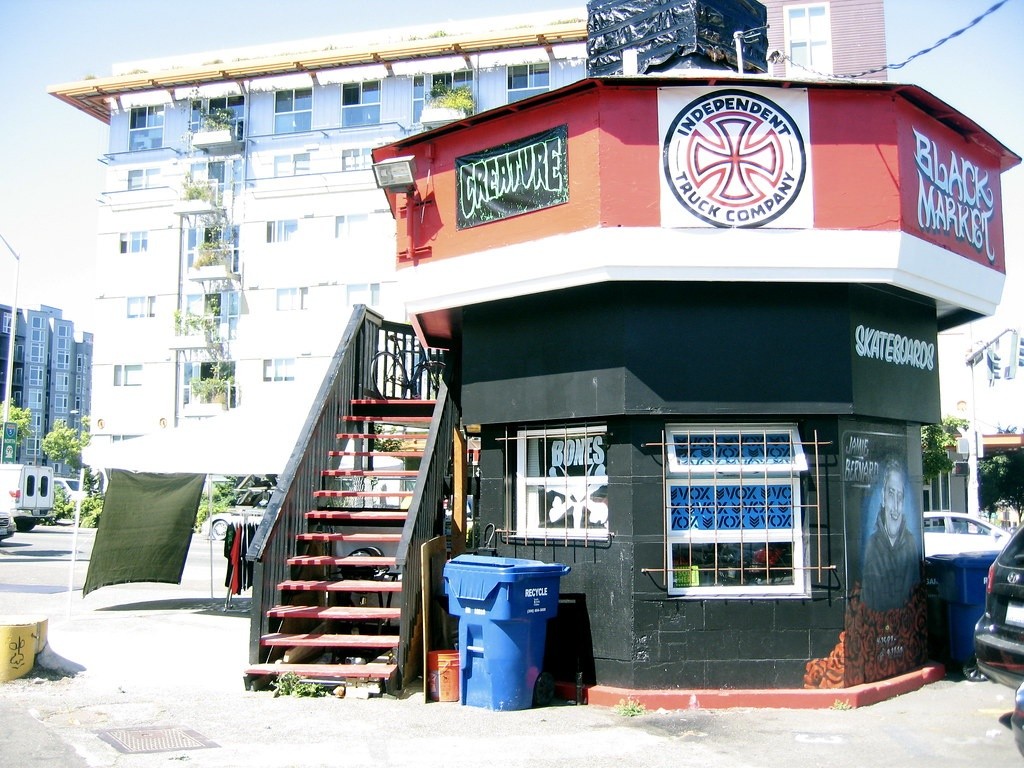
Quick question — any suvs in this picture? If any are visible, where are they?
[199,486,278,541]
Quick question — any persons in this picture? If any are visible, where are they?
[859,459,921,614]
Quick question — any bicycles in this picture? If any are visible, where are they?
[369,333,445,400]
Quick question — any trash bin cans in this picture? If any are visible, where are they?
[922,550,1001,665]
[442,552,572,712]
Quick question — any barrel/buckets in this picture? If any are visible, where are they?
[428,649,459,702]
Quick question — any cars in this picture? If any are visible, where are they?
[923,510,1013,557]
[973,520,1023,691]
[0,510,17,544]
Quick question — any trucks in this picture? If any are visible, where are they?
[0,461,55,532]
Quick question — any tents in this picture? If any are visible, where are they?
[68,375,323,613]
